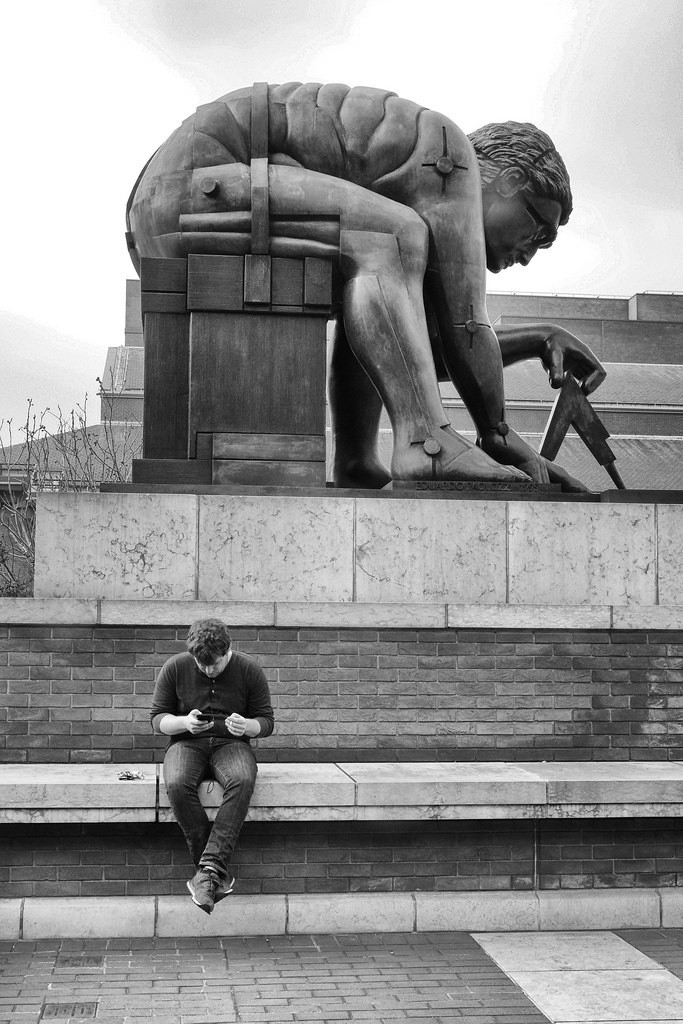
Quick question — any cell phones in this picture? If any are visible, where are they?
[197,714,227,720]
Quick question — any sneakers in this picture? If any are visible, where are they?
[186,869,220,915]
[214,872,235,903]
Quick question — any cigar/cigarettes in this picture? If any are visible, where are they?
[230,720,232,726]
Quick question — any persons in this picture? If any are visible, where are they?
[149,619,273,915]
[124,79,605,481]
